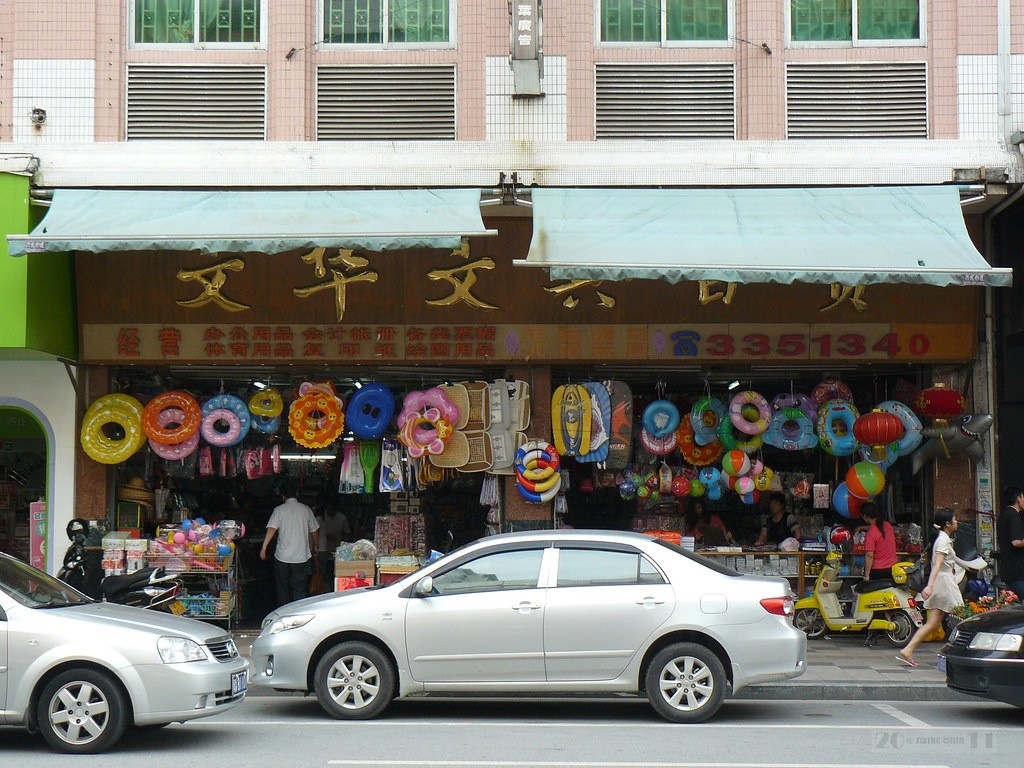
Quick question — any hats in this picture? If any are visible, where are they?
[116,477,154,502]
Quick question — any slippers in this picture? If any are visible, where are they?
[895,656,919,667]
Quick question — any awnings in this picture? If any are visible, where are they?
[510,188,1014,289]
[5,188,497,257]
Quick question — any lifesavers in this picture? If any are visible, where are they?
[675,396,728,466]
[811,379,861,457]
[141,391,201,460]
[344,383,395,440]
[858,400,924,468]
[80,393,146,465]
[247,387,284,434]
[396,386,461,459]
[761,393,819,451]
[287,379,346,450]
[514,440,562,503]
[199,394,251,447]
[719,390,772,453]
[639,399,680,455]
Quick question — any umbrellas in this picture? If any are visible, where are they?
[156,480,170,518]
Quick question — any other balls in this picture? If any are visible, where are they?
[832,461,885,520]
[619,449,773,505]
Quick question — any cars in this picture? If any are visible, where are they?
[0,549,248,754]
[936,607,1024,714]
[247,531,807,723]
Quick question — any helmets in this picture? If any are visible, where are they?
[831,527,853,544]
[67,518,90,541]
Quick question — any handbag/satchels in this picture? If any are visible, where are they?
[308,567,322,594]
[907,544,931,592]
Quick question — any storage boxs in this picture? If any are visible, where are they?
[334,559,376,577]
[100,530,152,577]
[377,565,420,585]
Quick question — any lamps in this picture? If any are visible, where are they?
[352,378,364,391]
[726,377,742,390]
[252,379,270,389]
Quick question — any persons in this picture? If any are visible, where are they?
[860,502,898,622]
[259,482,320,605]
[324,501,348,590]
[314,508,326,580]
[755,491,801,545]
[685,499,732,540]
[996,486,1024,601]
[895,511,979,667]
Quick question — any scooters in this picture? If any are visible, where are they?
[59,518,187,618]
[795,550,916,646]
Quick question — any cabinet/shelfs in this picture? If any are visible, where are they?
[696,549,924,603]
[144,545,236,630]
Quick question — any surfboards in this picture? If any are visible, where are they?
[550,380,634,470]
